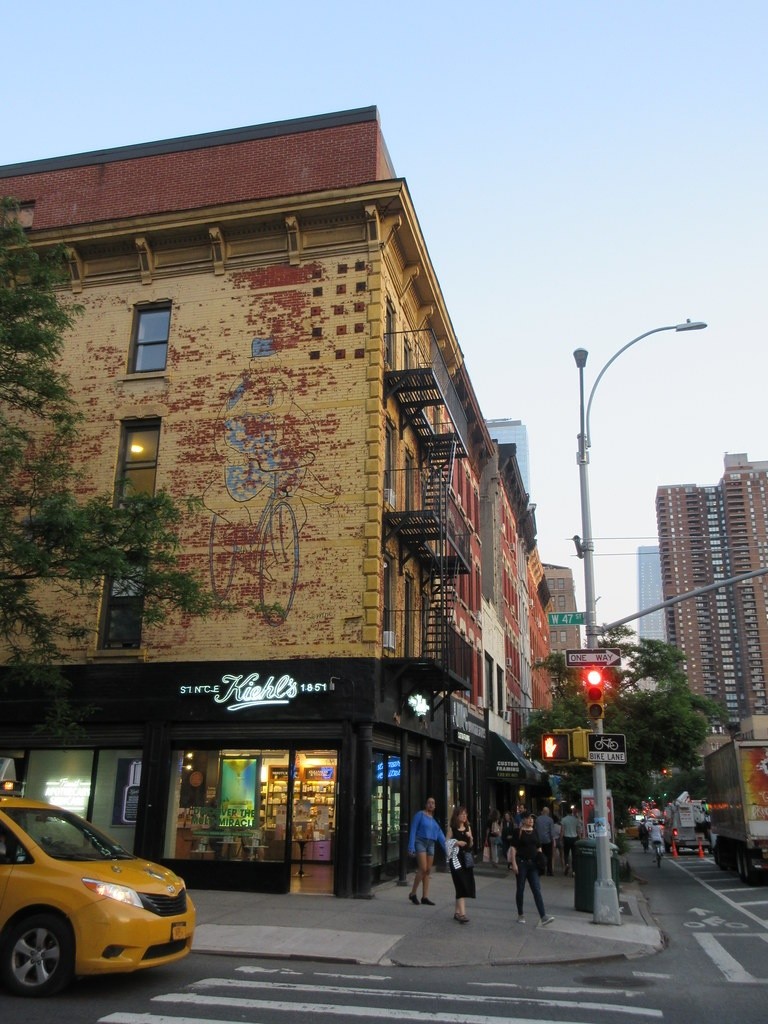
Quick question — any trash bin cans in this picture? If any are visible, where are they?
[573,840,620,913]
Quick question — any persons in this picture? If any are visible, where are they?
[444,806,476,922]
[510,812,555,925]
[407,796,446,906]
[638,818,666,859]
[484,803,583,878]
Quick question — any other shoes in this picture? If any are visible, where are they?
[454,913,470,922]
[409,892,421,905]
[421,897,435,905]
[541,916,554,925]
[518,915,526,924]
[565,864,568,875]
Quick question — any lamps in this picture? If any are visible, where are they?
[328,676,341,691]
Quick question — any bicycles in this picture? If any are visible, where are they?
[651,837,664,868]
[639,832,649,854]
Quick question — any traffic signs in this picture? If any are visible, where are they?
[564,648,623,667]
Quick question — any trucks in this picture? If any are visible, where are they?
[704,730,768,885]
[662,791,716,854]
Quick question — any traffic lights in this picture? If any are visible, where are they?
[585,667,607,720]
[543,734,571,761]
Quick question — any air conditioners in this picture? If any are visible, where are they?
[529,599,533,606]
[543,636,546,641]
[509,543,514,550]
[503,711,512,724]
[537,621,542,628]
[383,489,395,510]
[505,658,512,667]
[382,630,396,650]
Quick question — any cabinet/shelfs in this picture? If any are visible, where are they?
[265,765,336,839]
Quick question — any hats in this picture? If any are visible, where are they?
[520,810,537,819]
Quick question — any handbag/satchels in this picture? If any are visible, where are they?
[506,846,518,871]
[483,843,490,863]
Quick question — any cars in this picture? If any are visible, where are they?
[0,757,197,1000]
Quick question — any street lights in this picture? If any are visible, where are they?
[580,316,711,928]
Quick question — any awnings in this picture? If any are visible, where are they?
[488,731,542,786]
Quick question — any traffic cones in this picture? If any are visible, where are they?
[697,840,705,858]
[671,839,679,857]
